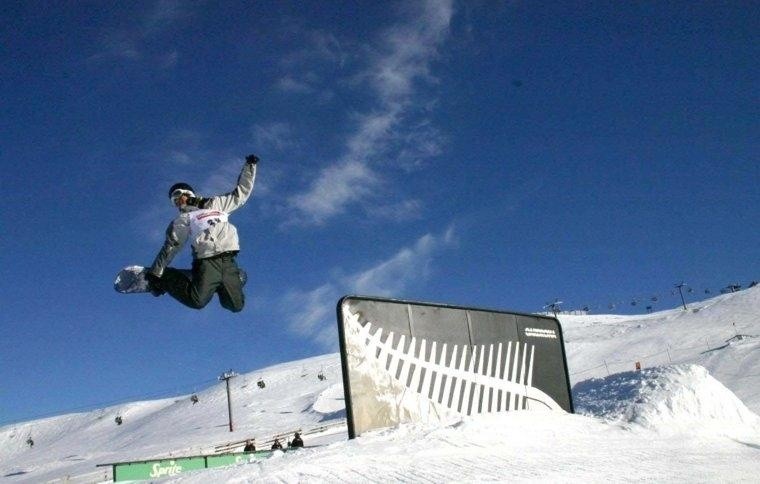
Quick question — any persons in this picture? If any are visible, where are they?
[271,436,283,448]
[243,439,256,451]
[291,431,304,447]
[111,151,259,312]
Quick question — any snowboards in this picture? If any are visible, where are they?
[114,266,247,294]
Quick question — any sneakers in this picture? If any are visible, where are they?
[150,285,165,299]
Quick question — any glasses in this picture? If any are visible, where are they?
[170,188,183,206]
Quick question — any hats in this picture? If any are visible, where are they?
[168,181,195,200]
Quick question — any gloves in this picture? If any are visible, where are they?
[245,153,261,164]
[144,271,161,286]
